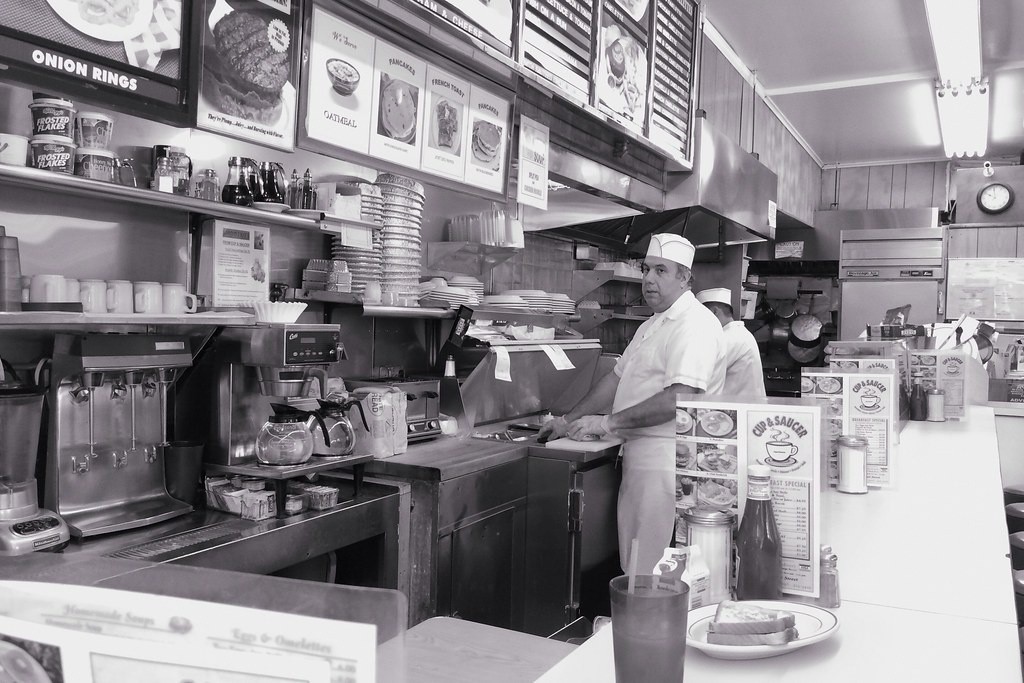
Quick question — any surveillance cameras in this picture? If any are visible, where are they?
[984,161,991,168]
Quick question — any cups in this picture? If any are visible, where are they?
[62,279,197,317]
[609,574,690,683]
[28,275,67,302]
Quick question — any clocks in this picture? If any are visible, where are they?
[976,181,1015,214]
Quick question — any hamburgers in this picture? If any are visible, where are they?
[675,444,690,467]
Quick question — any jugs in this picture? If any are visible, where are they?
[223,155,291,207]
[306,396,370,456]
[255,401,330,465]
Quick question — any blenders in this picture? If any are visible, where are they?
[0,380,72,559]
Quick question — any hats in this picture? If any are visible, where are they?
[647,233,696,270]
[696,288,732,305]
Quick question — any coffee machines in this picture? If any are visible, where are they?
[176,310,376,516]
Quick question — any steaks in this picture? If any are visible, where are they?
[215,11,291,95]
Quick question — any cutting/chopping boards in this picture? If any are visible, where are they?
[546,433,625,452]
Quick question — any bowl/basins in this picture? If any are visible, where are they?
[326,58,360,96]
[0,96,119,183]
[252,301,307,324]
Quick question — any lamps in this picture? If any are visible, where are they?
[923,0,989,156]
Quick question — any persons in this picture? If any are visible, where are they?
[697,286,768,399]
[536,233,727,578]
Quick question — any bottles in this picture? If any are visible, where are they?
[152,157,176,194]
[684,373,945,609]
[287,167,319,211]
[199,170,221,204]
[170,147,193,195]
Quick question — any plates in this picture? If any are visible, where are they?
[683,601,839,662]
[46,0,153,43]
[336,171,577,315]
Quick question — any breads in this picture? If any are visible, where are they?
[706,599,799,647]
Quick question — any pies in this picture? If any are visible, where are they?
[380,81,417,139]
[472,121,501,162]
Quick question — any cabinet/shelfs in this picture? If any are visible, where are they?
[0,161,581,323]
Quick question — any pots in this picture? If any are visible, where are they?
[757,290,823,365]
